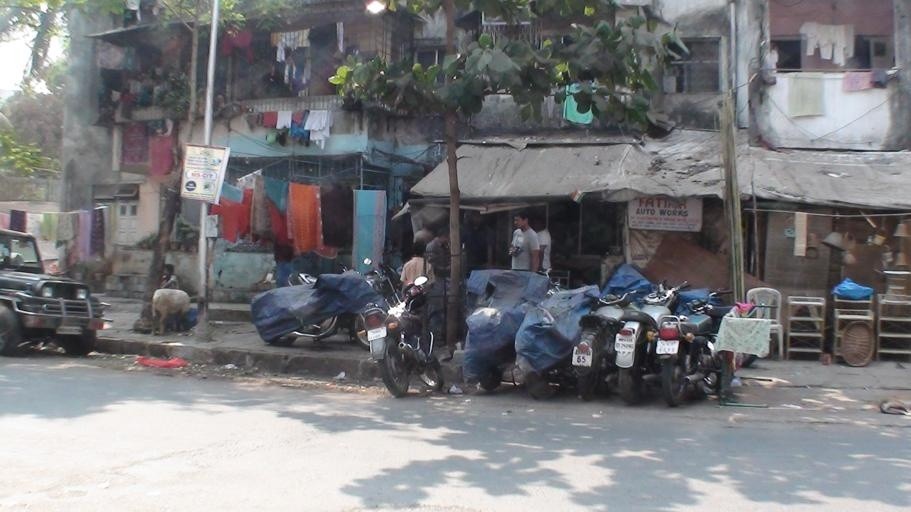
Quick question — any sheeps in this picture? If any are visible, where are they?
[150,288,197,335]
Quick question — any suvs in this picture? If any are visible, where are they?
[0,228,108,360]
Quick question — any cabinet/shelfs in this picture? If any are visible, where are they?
[876,293,911,359]
[786,297,825,359]
[833,289,873,357]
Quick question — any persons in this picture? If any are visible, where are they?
[509,211,540,272]
[160,264,180,289]
[413,230,451,343]
[532,215,552,277]
[274,244,293,288]
[400,241,436,326]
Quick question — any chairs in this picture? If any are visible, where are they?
[747,287,783,362]
